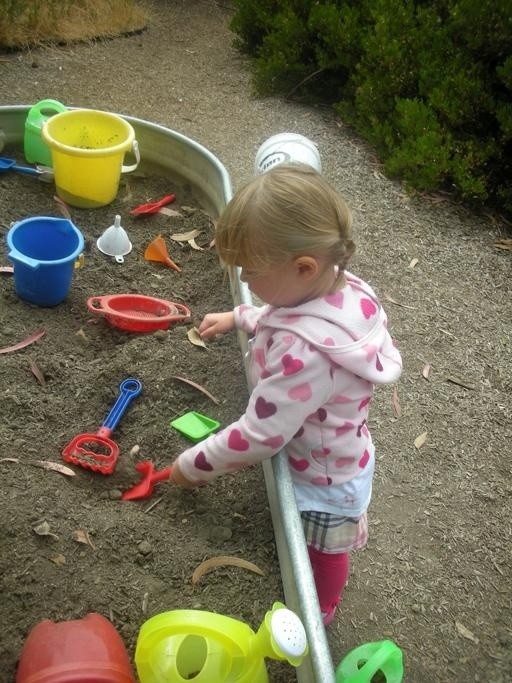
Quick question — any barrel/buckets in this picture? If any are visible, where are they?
[41,109,141,209]
[255,131,323,174]
[6,216,85,308]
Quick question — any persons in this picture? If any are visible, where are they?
[168,159,406,633]
